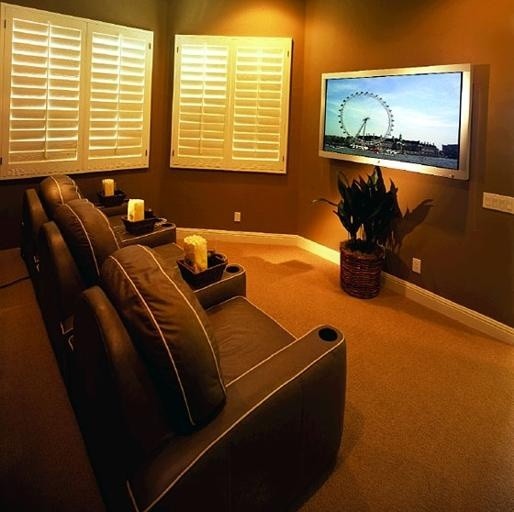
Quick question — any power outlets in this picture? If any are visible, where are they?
[412,257,423,275]
[234,212,242,222]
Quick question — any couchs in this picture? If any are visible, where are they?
[1,173,350,511]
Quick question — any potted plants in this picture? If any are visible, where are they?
[310,164,405,300]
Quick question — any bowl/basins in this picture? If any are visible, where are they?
[97,190,126,207]
[121,211,158,233]
[177,252,228,286]
